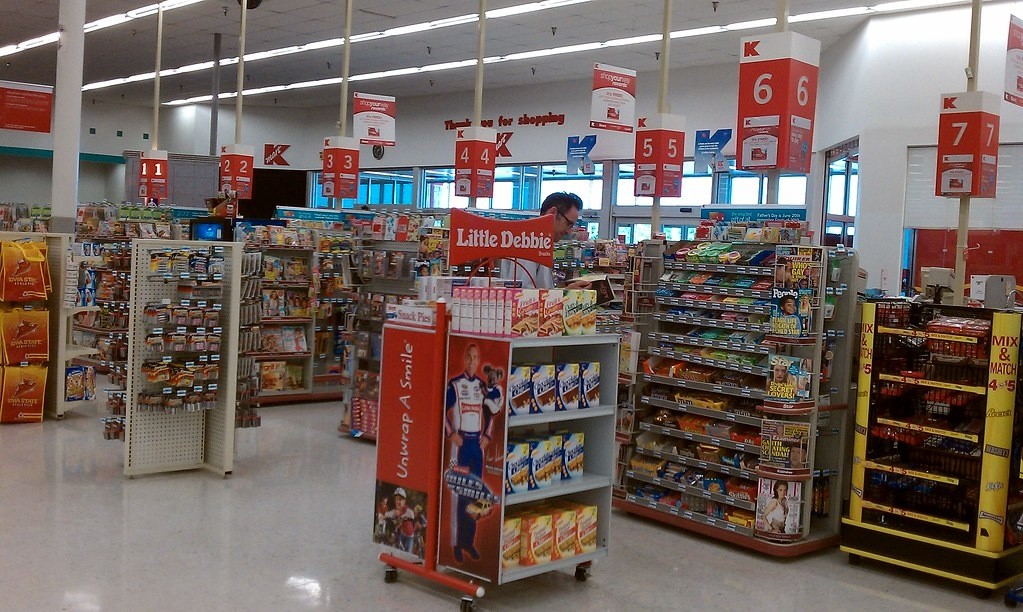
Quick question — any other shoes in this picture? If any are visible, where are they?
[454,547,463,562]
[464,544,479,560]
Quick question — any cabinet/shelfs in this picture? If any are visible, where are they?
[550,240,663,444]
[236,222,360,405]
[335,203,584,445]
[86,233,264,478]
[612,241,859,559]
[839,301,1023,600]
[1,230,102,422]
[369,317,439,569]
[434,329,622,586]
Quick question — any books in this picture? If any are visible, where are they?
[562,275,616,306]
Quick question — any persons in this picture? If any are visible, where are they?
[420,234,444,277]
[801,359,809,371]
[779,295,801,338]
[785,444,807,468]
[773,425,783,441]
[263,252,309,390]
[777,256,820,289]
[798,296,813,331]
[445,345,504,563]
[799,376,810,390]
[376,487,416,554]
[500,193,593,289]
[761,480,789,534]
[766,357,797,399]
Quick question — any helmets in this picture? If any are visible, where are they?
[394,487,406,498]
[414,505,422,513]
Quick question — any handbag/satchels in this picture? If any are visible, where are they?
[0,241,51,422]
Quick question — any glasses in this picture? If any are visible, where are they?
[557,210,575,229]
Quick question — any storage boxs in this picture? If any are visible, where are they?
[446,286,599,574]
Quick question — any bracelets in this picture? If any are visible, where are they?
[484,433,491,441]
[448,430,454,438]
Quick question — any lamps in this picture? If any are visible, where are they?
[0,0,1023,107]
